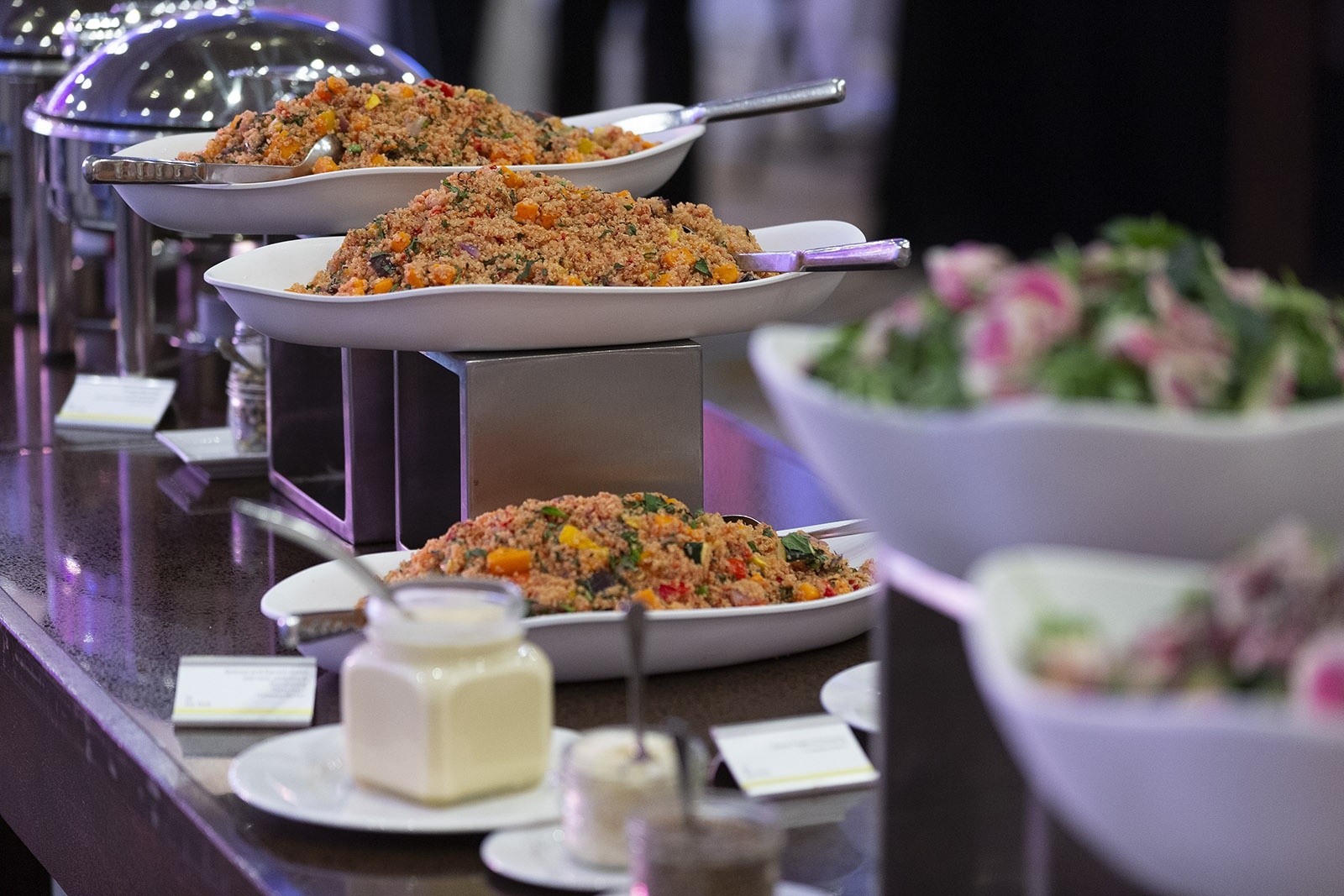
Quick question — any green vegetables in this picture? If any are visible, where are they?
[255,110,568,159]
[538,492,823,613]
[376,179,713,287]
[808,217,1344,415]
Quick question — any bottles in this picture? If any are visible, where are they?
[341,578,553,804]
[227,319,267,454]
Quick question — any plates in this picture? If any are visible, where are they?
[820,660,884,735]
[483,800,640,890]
[172,655,317,728]
[109,103,707,233]
[711,714,880,799]
[54,374,177,431]
[260,549,885,679]
[608,879,828,896]
[155,426,268,466]
[227,722,581,834]
[203,220,867,352]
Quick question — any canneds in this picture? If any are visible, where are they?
[559,726,707,870]
[339,575,555,805]
[624,793,785,896]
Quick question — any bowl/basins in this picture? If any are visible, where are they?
[634,800,781,896]
[23,102,191,234]
[964,546,1344,896]
[561,726,704,869]
[748,323,1344,592]
